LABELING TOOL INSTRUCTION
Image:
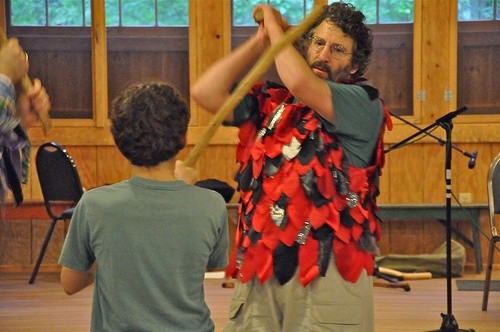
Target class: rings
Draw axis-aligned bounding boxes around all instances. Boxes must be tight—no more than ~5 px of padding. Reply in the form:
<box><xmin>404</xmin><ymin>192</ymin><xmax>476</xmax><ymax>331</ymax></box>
<box><xmin>24</xmin><ymin>52</ymin><xmax>28</xmax><ymax>62</ymax></box>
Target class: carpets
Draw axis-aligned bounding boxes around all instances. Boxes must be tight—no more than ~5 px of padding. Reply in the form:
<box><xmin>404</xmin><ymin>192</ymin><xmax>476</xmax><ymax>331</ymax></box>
<box><xmin>457</xmin><ymin>279</ymin><xmax>500</xmax><ymax>291</ymax></box>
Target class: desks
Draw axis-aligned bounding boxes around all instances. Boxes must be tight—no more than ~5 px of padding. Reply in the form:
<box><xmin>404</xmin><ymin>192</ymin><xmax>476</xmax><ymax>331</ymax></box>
<box><xmin>377</xmin><ymin>203</ymin><xmax>488</xmax><ymax>274</ymax></box>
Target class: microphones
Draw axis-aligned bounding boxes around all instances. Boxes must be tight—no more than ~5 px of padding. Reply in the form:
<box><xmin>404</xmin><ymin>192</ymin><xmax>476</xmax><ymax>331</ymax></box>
<box><xmin>469</xmin><ymin>150</ymin><xmax>478</xmax><ymax>168</ymax></box>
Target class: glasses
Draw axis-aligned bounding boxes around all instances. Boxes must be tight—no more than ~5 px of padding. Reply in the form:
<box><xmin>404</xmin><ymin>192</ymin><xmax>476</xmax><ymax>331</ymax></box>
<box><xmin>308</xmin><ymin>35</ymin><xmax>353</xmax><ymax>59</ymax></box>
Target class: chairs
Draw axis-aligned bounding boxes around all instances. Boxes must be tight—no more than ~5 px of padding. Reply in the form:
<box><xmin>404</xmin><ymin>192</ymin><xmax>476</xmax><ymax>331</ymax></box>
<box><xmin>29</xmin><ymin>141</ymin><xmax>83</xmax><ymax>284</ymax></box>
<box><xmin>480</xmin><ymin>153</ymin><xmax>500</xmax><ymax>312</ymax></box>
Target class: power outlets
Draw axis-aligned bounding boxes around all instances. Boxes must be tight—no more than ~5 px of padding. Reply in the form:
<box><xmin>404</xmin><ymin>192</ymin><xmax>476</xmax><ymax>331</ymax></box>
<box><xmin>458</xmin><ymin>193</ymin><xmax>471</xmax><ymax>204</ymax></box>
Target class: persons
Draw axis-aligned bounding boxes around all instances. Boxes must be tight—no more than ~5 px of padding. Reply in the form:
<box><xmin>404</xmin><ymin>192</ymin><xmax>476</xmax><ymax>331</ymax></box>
<box><xmin>58</xmin><ymin>81</ymin><xmax>229</xmax><ymax>332</ymax></box>
<box><xmin>190</xmin><ymin>1</ymin><xmax>393</xmax><ymax>332</ymax></box>
<box><xmin>0</xmin><ymin>37</ymin><xmax>51</xmax><ymax>208</ymax></box>
